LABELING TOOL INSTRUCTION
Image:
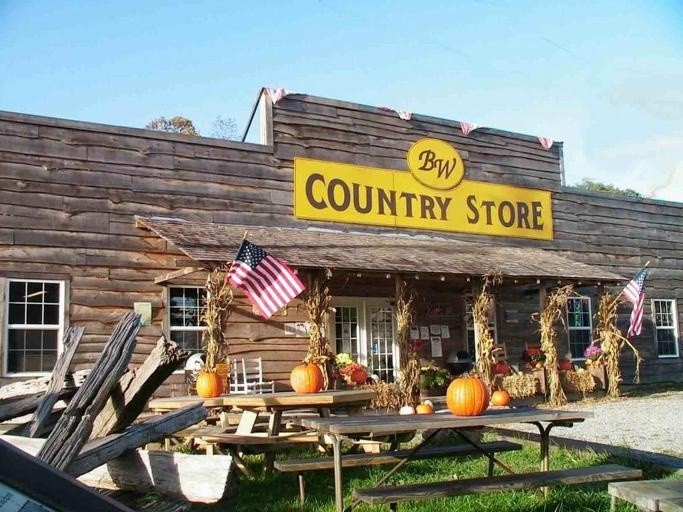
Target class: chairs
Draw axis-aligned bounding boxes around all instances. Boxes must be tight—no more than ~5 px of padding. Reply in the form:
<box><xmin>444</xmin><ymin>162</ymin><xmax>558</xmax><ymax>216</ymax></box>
<box><xmin>229</xmin><ymin>356</ymin><xmax>275</xmax><ymax>395</ymax></box>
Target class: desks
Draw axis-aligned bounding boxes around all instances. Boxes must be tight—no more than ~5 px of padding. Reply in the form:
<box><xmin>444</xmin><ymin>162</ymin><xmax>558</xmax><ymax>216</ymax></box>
<box><xmin>149</xmin><ymin>391</ymin><xmax>378</xmax><ymax>457</ymax></box>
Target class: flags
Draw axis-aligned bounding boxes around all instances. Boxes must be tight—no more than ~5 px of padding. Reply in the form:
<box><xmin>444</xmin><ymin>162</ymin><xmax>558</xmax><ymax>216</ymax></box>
<box><xmin>619</xmin><ymin>266</ymin><xmax>648</xmax><ymax>338</ymax></box>
<box><xmin>225</xmin><ymin>238</ymin><xmax>307</xmax><ymax>323</ymax></box>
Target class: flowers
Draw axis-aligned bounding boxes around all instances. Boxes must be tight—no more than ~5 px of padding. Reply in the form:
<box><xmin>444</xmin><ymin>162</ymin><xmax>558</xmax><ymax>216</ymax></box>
<box><xmin>527</xmin><ymin>346</ymin><xmax>545</xmax><ymax>362</ymax></box>
<box><xmin>584</xmin><ymin>345</ymin><xmax>601</xmax><ymax>361</ymax></box>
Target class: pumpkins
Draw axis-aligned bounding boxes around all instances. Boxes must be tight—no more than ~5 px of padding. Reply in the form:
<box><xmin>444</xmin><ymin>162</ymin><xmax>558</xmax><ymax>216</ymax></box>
<box><xmin>446</xmin><ymin>371</ymin><xmax>489</xmax><ymax>416</ymax></box>
<box><xmin>491</xmin><ymin>388</ymin><xmax>511</xmax><ymax>406</ymax></box>
<box><xmin>289</xmin><ymin>359</ymin><xmax>323</xmax><ymax>393</ymax></box>
<box><xmin>196</xmin><ymin>368</ymin><xmax>224</xmax><ymax>398</ymax></box>
<box><xmin>416</xmin><ymin>404</ymin><xmax>433</xmax><ymax>414</ymax></box>
<box><xmin>399</xmin><ymin>404</ymin><xmax>415</xmax><ymax>415</ymax></box>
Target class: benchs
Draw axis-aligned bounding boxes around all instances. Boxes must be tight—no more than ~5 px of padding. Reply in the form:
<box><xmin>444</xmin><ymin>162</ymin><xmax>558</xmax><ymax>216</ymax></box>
<box><xmin>272</xmin><ymin>440</ymin><xmax>644</xmax><ymax>507</ymax></box>
<box><xmin>163</xmin><ymin>410</ymin><xmax>354</xmax><ymax>446</ymax></box>
